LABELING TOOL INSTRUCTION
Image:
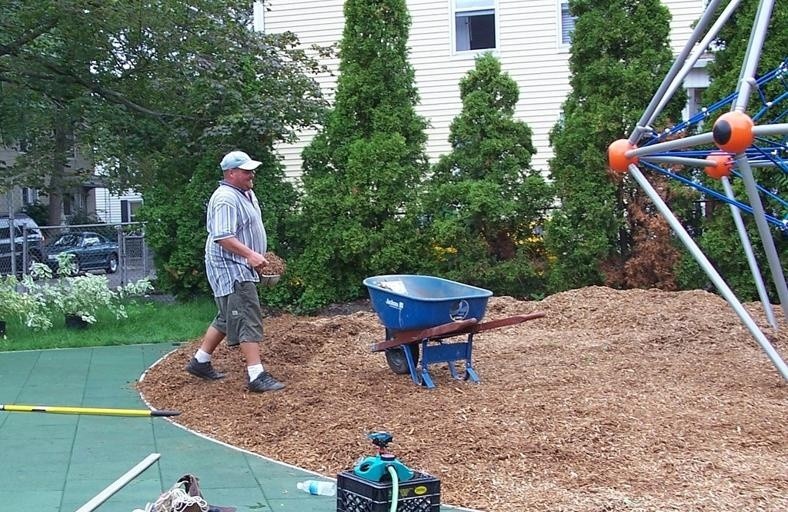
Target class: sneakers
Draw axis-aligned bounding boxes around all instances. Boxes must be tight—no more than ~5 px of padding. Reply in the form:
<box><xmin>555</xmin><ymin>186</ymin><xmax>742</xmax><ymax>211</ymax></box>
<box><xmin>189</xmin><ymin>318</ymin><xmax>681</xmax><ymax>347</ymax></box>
<box><xmin>248</xmin><ymin>370</ymin><xmax>286</xmax><ymax>392</ymax></box>
<box><xmin>184</xmin><ymin>356</ymin><xmax>224</xmax><ymax>381</ymax></box>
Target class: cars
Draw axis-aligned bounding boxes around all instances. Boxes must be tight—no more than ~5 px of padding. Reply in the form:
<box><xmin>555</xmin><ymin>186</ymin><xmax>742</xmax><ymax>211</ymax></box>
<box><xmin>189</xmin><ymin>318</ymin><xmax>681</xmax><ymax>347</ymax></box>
<box><xmin>0</xmin><ymin>213</ymin><xmax>119</xmax><ymax>281</ymax></box>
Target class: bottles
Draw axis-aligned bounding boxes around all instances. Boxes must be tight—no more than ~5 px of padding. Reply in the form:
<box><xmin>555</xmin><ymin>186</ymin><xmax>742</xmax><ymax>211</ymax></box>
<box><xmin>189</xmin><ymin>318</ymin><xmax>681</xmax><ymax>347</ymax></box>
<box><xmin>296</xmin><ymin>480</ymin><xmax>337</xmax><ymax>497</ymax></box>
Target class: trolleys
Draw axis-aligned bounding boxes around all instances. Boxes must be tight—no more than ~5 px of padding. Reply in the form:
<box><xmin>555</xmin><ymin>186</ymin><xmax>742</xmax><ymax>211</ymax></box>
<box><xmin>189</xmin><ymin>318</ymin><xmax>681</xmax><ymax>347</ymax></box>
<box><xmin>363</xmin><ymin>274</ymin><xmax>546</xmax><ymax>389</ymax></box>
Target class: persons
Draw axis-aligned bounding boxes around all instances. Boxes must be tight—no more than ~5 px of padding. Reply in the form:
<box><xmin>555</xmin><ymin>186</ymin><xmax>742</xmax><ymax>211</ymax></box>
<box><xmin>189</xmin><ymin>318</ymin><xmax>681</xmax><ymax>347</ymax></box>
<box><xmin>181</xmin><ymin>150</ymin><xmax>288</xmax><ymax>392</ymax></box>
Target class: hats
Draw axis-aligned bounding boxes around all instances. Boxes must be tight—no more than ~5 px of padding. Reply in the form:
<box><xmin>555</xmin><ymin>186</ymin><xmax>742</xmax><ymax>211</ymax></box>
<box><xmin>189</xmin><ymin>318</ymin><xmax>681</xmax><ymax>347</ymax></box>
<box><xmin>220</xmin><ymin>150</ymin><xmax>264</xmax><ymax>172</ymax></box>
